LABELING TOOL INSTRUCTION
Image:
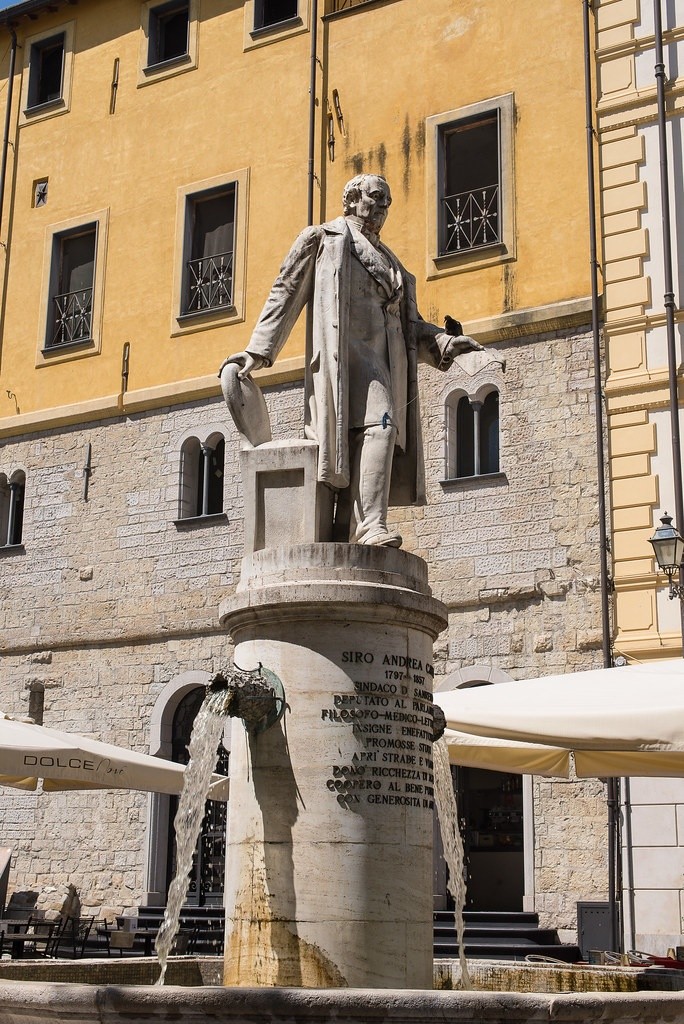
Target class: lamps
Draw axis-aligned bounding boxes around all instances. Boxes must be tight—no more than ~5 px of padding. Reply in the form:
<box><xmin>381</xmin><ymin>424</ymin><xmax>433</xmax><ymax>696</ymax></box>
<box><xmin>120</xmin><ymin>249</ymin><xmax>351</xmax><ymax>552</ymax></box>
<box><xmin>646</xmin><ymin>511</ymin><xmax>684</xmax><ymax>602</ymax></box>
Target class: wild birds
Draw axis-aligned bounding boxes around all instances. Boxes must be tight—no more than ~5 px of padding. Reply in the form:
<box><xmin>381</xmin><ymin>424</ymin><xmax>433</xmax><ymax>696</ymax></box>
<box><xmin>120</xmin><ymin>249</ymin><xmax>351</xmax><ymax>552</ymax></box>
<box><xmin>443</xmin><ymin>315</ymin><xmax>462</xmax><ymax>337</ymax></box>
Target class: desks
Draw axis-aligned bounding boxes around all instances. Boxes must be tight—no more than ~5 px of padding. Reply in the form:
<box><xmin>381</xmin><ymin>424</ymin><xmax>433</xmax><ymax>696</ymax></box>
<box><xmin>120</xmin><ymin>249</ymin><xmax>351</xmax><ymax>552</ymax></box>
<box><xmin>0</xmin><ymin>934</ymin><xmax>60</xmax><ymax>959</ymax></box>
<box><xmin>96</xmin><ymin>928</ymin><xmax>160</xmax><ymax>958</ymax></box>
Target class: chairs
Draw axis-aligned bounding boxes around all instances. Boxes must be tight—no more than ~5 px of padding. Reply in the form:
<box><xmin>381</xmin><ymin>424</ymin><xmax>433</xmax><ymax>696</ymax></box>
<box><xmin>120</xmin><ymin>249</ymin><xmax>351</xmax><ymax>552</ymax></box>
<box><xmin>627</xmin><ymin>950</ymin><xmax>656</xmax><ymax>966</ymax></box>
<box><xmin>604</xmin><ymin>950</ymin><xmax>639</xmax><ymax>966</ymax></box>
<box><xmin>21</xmin><ymin>916</ymin><xmax>63</xmax><ymax>960</ymax></box>
<box><xmin>525</xmin><ymin>954</ymin><xmax>570</xmax><ymax>965</ymax></box>
<box><xmin>169</xmin><ymin>926</ymin><xmax>200</xmax><ymax>955</ymax></box>
<box><xmin>72</xmin><ymin>918</ymin><xmax>110</xmax><ymax>959</ymax></box>
<box><xmin>54</xmin><ymin>916</ymin><xmax>94</xmax><ymax>959</ymax></box>
